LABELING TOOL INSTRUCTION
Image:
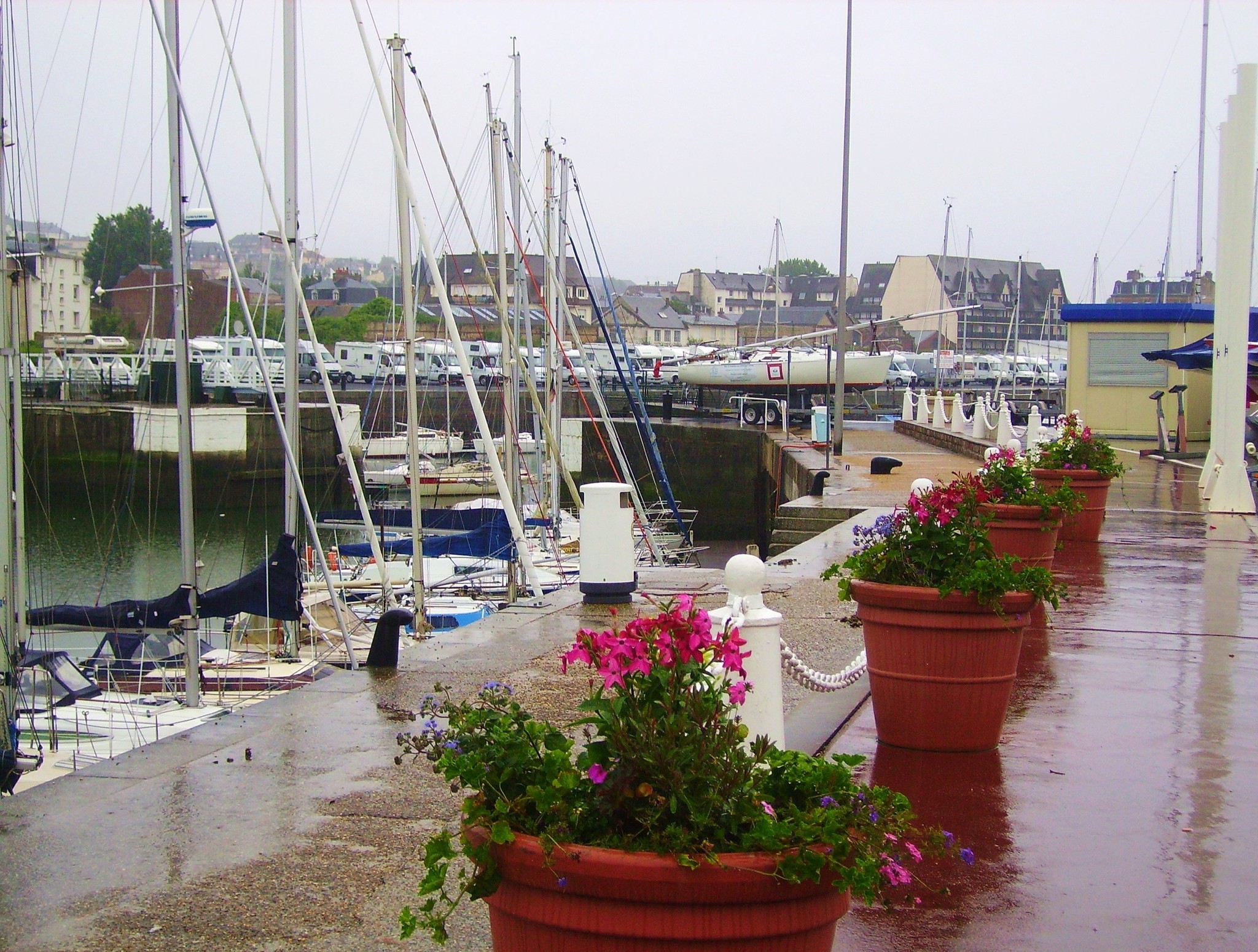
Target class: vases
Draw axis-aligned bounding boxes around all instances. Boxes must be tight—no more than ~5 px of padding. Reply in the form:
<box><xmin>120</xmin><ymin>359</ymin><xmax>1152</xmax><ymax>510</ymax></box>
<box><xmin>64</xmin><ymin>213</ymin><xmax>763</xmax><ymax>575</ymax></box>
<box><xmin>848</xmin><ymin>576</ymin><xmax>1037</xmax><ymax>751</ymax></box>
<box><xmin>975</xmin><ymin>498</ymin><xmax>1061</xmax><ymax>588</ymax></box>
<box><xmin>1032</xmin><ymin>462</ymin><xmax>1111</xmax><ymax>546</ymax></box>
<box><xmin>460</xmin><ymin>794</ymin><xmax>852</xmax><ymax>952</ymax></box>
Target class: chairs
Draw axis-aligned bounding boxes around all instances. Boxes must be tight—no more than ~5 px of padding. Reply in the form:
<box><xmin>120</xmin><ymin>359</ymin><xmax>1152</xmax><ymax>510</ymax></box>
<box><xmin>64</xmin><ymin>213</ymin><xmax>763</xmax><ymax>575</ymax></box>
<box><xmin>1008</xmin><ymin>400</ymin><xmax>1028</xmax><ymax>426</ymax></box>
<box><xmin>1038</xmin><ymin>401</ymin><xmax>1059</xmax><ymax>428</ymax></box>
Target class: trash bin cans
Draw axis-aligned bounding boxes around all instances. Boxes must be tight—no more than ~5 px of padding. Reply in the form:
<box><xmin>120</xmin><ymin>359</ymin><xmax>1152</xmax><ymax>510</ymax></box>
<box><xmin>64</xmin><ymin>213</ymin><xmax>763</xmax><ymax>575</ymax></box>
<box><xmin>811</xmin><ymin>406</ymin><xmax>830</xmax><ymax>444</ymax></box>
<box><xmin>579</xmin><ymin>483</ymin><xmax>639</xmax><ymax>603</ymax></box>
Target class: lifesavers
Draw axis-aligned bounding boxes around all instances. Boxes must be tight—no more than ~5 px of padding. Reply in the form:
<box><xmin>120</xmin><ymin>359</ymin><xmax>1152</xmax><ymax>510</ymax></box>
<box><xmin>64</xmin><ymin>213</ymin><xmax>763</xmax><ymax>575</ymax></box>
<box><xmin>305</xmin><ymin>546</ymin><xmax>312</xmax><ymax>572</ymax></box>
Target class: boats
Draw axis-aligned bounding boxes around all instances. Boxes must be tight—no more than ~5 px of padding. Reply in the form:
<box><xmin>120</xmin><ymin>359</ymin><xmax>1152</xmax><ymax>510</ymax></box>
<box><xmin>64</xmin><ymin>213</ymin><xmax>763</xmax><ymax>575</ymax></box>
<box><xmin>675</xmin><ymin>218</ymin><xmax>900</xmax><ymax>396</ymax></box>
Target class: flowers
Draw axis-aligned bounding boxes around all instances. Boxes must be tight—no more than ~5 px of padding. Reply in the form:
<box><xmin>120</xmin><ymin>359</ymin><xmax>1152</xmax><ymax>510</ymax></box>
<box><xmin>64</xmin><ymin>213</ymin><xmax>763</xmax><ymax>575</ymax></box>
<box><xmin>388</xmin><ymin>594</ymin><xmax>971</xmax><ymax>931</ymax></box>
<box><xmin>1034</xmin><ymin>414</ymin><xmax>1138</xmax><ymax>518</ymax></box>
<box><xmin>817</xmin><ymin>479</ymin><xmax>1060</xmax><ymax>612</ymax></box>
<box><xmin>955</xmin><ymin>453</ymin><xmax>1087</xmax><ymax>514</ymax></box>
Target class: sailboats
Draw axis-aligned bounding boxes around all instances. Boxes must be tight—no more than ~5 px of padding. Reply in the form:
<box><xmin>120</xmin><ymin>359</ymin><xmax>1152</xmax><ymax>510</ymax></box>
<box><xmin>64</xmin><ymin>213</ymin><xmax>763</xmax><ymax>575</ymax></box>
<box><xmin>0</xmin><ymin>0</ymin><xmax>1067</xmax><ymax>808</ymax></box>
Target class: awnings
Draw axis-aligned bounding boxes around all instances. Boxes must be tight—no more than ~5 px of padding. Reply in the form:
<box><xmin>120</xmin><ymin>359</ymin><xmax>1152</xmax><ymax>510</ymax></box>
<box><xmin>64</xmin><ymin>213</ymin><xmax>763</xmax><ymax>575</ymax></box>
<box><xmin>1141</xmin><ymin>312</ymin><xmax>1258</xmax><ymax>370</ymax></box>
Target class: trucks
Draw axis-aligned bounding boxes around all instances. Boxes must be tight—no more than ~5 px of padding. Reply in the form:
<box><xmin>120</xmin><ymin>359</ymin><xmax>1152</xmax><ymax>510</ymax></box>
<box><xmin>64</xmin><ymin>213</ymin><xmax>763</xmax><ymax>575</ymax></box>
<box><xmin>972</xmin><ymin>355</ymin><xmax>1006</xmax><ymax>386</ymax></box>
<box><xmin>461</xmin><ymin>339</ymin><xmax>508</xmax><ymax>386</ymax></box>
<box><xmin>276</xmin><ymin>339</ymin><xmax>343</xmax><ymax>385</ymax></box>
<box><xmin>191</xmin><ymin>334</ymin><xmax>286</xmax><ymax>388</ymax></box>
<box><xmin>415</xmin><ymin>338</ymin><xmax>465</xmax><ymax>386</ymax></box>
<box><xmin>514</xmin><ymin>346</ymin><xmax>548</xmax><ymax>388</ymax></box>
<box><xmin>557</xmin><ymin>340</ymin><xmax>597</xmax><ymax>387</ymax></box>
<box><xmin>997</xmin><ymin>356</ymin><xmax>1035</xmax><ymax>386</ymax></box>
<box><xmin>1025</xmin><ymin>357</ymin><xmax>1059</xmax><ymax>385</ymax></box>
<box><xmin>142</xmin><ymin>336</ymin><xmax>234</xmax><ymax>384</ymax></box>
<box><xmin>582</xmin><ymin>342</ymin><xmax>664</xmax><ymax>387</ymax></box>
<box><xmin>1045</xmin><ymin>358</ymin><xmax>1067</xmax><ymax>383</ymax></box>
<box><xmin>44</xmin><ymin>328</ymin><xmax>137</xmax><ymax>387</ymax></box>
<box><xmin>333</xmin><ymin>340</ymin><xmax>419</xmax><ymax>386</ymax></box>
